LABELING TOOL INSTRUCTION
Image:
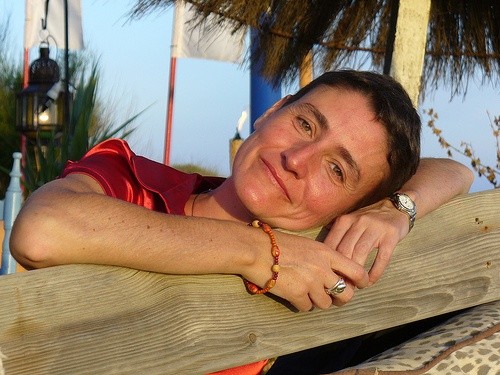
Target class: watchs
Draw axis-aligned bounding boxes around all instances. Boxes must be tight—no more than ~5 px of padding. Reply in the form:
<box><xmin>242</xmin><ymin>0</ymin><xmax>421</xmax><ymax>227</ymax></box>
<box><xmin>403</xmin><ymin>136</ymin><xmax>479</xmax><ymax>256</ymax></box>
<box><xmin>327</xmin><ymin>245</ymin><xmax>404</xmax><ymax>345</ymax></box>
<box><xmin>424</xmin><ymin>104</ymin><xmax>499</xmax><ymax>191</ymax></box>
<box><xmin>389</xmin><ymin>191</ymin><xmax>416</xmax><ymax>232</ymax></box>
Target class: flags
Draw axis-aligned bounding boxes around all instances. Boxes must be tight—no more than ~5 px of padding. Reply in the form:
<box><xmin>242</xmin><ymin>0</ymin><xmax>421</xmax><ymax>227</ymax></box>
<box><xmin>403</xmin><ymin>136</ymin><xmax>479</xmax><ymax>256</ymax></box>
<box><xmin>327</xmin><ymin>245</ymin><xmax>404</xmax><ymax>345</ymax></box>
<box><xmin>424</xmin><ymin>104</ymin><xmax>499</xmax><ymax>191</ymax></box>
<box><xmin>177</xmin><ymin>0</ymin><xmax>244</xmax><ymax>62</ymax></box>
<box><xmin>26</xmin><ymin>0</ymin><xmax>84</xmax><ymax>51</ymax></box>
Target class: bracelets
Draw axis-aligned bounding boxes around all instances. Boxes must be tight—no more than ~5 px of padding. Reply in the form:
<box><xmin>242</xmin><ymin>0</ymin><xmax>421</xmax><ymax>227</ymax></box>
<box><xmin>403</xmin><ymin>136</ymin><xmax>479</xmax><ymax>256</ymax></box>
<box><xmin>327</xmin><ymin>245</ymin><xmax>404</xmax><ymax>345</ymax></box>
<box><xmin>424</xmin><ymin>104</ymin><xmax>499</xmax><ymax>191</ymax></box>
<box><xmin>242</xmin><ymin>220</ymin><xmax>280</xmax><ymax>295</ymax></box>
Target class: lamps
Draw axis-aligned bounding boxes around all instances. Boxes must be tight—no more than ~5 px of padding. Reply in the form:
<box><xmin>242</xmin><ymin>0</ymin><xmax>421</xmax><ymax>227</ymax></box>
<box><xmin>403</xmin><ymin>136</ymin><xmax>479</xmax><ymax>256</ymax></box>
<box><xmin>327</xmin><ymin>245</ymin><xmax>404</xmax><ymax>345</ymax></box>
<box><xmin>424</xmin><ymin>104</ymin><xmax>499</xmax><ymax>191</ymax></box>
<box><xmin>14</xmin><ymin>0</ymin><xmax>74</xmax><ymax>146</ymax></box>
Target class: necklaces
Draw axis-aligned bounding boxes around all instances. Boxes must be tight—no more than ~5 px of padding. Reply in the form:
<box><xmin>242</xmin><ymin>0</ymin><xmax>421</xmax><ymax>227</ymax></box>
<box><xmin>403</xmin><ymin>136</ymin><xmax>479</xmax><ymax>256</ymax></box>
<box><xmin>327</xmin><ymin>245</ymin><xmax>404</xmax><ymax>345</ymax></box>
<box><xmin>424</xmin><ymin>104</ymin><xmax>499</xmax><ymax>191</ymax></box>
<box><xmin>192</xmin><ymin>188</ymin><xmax>213</xmax><ymax>216</ymax></box>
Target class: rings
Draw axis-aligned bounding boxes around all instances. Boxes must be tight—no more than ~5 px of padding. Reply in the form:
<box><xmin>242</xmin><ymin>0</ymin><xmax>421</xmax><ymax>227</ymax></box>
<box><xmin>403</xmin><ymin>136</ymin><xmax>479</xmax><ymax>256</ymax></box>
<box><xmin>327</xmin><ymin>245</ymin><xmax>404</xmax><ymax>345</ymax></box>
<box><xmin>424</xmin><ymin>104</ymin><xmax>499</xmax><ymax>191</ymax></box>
<box><xmin>325</xmin><ymin>276</ymin><xmax>346</xmax><ymax>295</ymax></box>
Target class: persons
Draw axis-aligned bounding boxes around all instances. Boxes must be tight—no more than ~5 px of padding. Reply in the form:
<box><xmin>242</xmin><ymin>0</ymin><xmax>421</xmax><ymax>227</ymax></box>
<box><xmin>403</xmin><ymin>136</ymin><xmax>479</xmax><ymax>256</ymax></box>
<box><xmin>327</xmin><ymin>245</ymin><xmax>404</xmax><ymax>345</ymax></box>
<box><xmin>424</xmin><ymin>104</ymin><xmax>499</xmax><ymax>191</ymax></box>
<box><xmin>10</xmin><ymin>70</ymin><xmax>474</xmax><ymax>375</ymax></box>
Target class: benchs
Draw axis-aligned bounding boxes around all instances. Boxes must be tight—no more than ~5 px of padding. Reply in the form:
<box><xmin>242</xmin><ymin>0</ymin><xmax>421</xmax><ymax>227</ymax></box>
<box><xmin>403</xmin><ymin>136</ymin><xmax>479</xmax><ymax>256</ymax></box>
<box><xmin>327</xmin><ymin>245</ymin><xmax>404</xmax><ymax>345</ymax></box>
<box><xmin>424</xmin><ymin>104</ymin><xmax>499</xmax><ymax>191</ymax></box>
<box><xmin>0</xmin><ymin>188</ymin><xmax>500</xmax><ymax>375</ymax></box>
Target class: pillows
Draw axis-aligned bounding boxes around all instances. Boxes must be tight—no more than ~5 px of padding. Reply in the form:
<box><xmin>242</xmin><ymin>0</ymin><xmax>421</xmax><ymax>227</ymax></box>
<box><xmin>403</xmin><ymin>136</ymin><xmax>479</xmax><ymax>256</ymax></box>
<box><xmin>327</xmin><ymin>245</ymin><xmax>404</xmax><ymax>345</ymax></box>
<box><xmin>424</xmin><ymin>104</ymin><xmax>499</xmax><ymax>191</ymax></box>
<box><xmin>323</xmin><ymin>301</ymin><xmax>500</xmax><ymax>375</ymax></box>
